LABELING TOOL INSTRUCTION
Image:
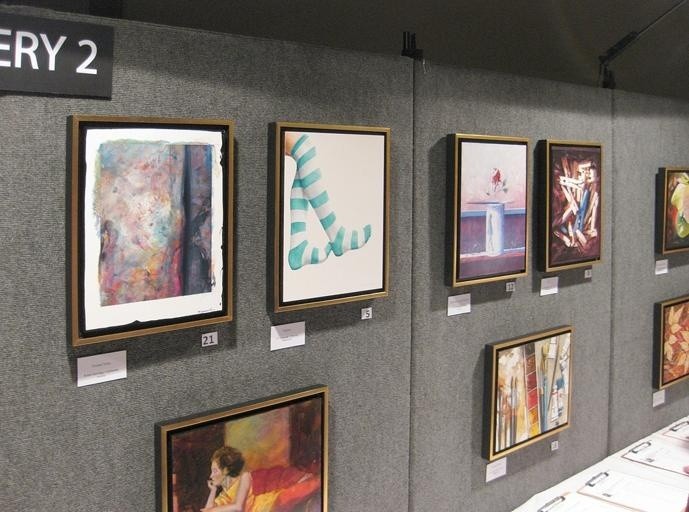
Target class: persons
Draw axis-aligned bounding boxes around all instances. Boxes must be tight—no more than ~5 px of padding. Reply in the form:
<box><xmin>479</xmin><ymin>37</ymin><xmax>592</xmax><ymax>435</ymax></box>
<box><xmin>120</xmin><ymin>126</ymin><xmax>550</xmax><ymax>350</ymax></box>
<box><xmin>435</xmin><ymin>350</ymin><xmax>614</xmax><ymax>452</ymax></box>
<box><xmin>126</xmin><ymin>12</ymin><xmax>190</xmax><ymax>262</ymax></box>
<box><xmin>282</xmin><ymin>129</ymin><xmax>372</xmax><ymax>271</ymax></box>
<box><xmin>199</xmin><ymin>444</ymin><xmax>279</xmax><ymax>512</ymax></box>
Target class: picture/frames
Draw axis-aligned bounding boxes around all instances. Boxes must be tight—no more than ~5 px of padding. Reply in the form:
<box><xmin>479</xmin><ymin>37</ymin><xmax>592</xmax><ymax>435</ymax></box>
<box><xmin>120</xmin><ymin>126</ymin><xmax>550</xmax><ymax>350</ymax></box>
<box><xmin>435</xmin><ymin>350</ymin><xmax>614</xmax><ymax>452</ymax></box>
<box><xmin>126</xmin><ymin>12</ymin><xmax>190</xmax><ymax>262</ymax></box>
<box><xmin>65</xmin><ymin>115</ymin><xmax>236</xmax><ymax>347</ymax></box>
<box><xmin>542</xmin><ymin>139</ymin><xmax>604</xmax><ymax>274</ymax></box>
<box><xmin>653</xmin><ymin>165</ymin><xmax>688</xmax><ymax>256</ymax></box>
<box><xmin>267</xmin><ymin>121</ymin><xmax>391</xmax><ymax>312</ymax></box>
<box><xmin>483</xmin><ymin>323</ymin><xmax>573</xmax><ymax>462</ymax></box>
<box><xmin>446</xmin><ymin>133</ymin><xmax>531</xmax><ymax>289</ymax></box>
<box><xmin>151</xmin><ymin>383</ymin><xmax>332</xmax><ymax>511</ymax></box>
<box><xmin>652</xmin><ymin>292</ymin><xmax>689</xmax><ymax>392</ymax></box>
